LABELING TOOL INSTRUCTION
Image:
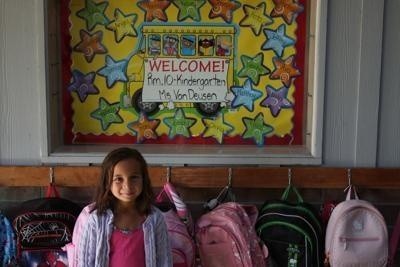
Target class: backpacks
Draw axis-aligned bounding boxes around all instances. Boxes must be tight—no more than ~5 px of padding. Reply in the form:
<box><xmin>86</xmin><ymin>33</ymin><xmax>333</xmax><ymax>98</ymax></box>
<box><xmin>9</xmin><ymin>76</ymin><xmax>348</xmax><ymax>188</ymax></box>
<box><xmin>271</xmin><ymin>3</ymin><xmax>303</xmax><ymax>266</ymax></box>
<box><xmin>153</xmin><ymin>182</ymin><xmax>200</xmax><ymax>267</ymax></box>
<box><xmin>11</xmin><ymin>184</ymin><xmax>81</xmax><ymax>267</ymax></box>
<box><xmin>193</xmin><ymin>184</ymin><xmax>274</xmax><ymax>267</ymax></box>
<box><xmin>256</xmin><ymin>182</ymin><xmax>325</xmax><ymax>267</ymax></box>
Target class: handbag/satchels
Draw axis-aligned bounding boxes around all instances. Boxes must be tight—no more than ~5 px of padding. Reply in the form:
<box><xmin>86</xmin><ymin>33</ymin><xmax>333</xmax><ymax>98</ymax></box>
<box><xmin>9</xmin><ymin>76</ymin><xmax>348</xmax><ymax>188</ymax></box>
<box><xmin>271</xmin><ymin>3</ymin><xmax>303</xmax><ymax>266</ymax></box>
<box><xmin>325</xmin><ymin>186</ymin><xmax>389</xmax><ymax>267</ymax></box>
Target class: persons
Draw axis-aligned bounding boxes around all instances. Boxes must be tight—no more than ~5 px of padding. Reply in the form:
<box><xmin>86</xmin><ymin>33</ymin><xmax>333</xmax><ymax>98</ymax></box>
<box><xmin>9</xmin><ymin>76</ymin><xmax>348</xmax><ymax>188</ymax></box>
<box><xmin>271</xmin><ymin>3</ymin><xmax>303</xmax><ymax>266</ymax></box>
<box><xmin>65</xmin><ymin>146</ymin><xmax>174</xmax><ymax>267</ymax></box>
